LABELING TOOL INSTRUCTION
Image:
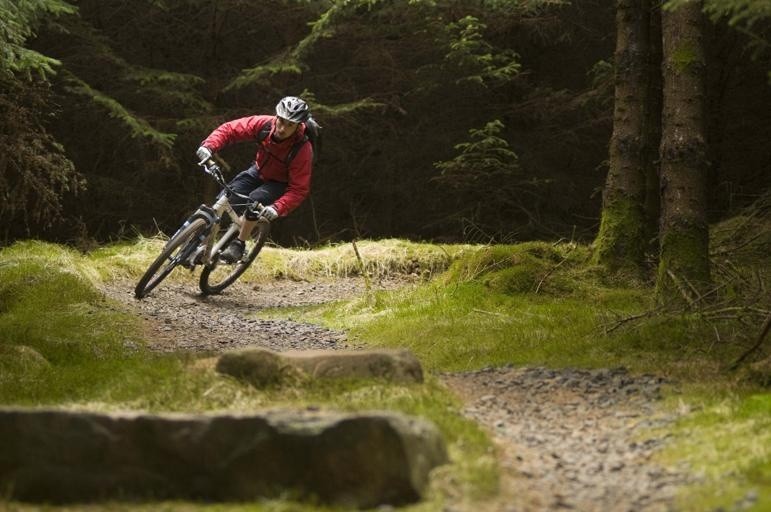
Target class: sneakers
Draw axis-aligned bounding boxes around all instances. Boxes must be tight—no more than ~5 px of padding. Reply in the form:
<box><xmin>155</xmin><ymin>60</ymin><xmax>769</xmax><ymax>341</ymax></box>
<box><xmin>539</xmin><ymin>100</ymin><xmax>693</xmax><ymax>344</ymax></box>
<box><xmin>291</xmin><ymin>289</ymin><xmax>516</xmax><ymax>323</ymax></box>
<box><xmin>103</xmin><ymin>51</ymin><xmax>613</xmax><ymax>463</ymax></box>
<box><xmin>221</xmin><ymin>240</ymin><xmax>246</xmax><ymax>263</ymax></box>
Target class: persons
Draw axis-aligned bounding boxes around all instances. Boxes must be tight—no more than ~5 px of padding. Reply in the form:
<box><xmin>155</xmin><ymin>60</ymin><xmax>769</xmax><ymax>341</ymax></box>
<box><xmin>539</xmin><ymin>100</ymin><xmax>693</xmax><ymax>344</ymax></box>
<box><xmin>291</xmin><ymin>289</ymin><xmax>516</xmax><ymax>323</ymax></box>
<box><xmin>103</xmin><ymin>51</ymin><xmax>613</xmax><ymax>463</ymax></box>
<box><xmin>186</xmin><ymin>95</ymin><xmax>313</xmax><ymax>266</ymax></box>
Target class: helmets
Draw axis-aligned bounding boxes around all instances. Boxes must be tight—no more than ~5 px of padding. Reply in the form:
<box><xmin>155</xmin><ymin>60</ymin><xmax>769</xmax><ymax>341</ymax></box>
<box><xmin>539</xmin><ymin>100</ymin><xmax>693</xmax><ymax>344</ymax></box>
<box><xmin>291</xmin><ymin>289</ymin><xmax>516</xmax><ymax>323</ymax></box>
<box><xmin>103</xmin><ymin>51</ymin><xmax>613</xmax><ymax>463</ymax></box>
<box><xmin>276</xmin><ymin>96</ymin><xmax>312</xmax><ymax>124</ymax></box>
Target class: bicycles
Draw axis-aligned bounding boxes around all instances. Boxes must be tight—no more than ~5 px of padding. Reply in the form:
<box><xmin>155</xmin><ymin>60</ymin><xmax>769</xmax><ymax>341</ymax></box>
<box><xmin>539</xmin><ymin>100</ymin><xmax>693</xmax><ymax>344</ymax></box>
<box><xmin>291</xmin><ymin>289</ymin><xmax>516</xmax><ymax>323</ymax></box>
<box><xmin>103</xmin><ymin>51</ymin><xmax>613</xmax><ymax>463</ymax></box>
<box><xmin>133</xmin><ymin>158</ymin><xmax>272</xmax><ymax>300</ymax></box>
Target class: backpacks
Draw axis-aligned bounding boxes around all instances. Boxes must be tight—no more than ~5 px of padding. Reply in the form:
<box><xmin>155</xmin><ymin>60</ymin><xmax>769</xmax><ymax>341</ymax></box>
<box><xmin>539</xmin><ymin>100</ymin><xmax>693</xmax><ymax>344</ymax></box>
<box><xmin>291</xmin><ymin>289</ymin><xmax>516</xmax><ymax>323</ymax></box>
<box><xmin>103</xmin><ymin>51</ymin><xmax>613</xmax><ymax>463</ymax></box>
<box><xmin>258</xmin><ymin>114</ymin><xmax>324</xmax><ymax>171</ymax></box>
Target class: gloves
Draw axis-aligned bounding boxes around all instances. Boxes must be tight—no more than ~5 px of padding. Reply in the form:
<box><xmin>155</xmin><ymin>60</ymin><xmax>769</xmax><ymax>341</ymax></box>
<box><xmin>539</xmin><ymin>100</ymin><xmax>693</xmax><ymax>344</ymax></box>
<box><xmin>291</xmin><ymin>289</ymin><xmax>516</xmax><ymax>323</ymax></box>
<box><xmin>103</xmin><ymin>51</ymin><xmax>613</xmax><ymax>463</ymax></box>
<box><xmin>195</xmin><ymin>146</ymin><xmax>214</xmax><ymax>167</ymax></box>
<box><xmin>257</xmin><ymin>206</ymin><xmax>280</xmax><ymax>223</ymax></box>
